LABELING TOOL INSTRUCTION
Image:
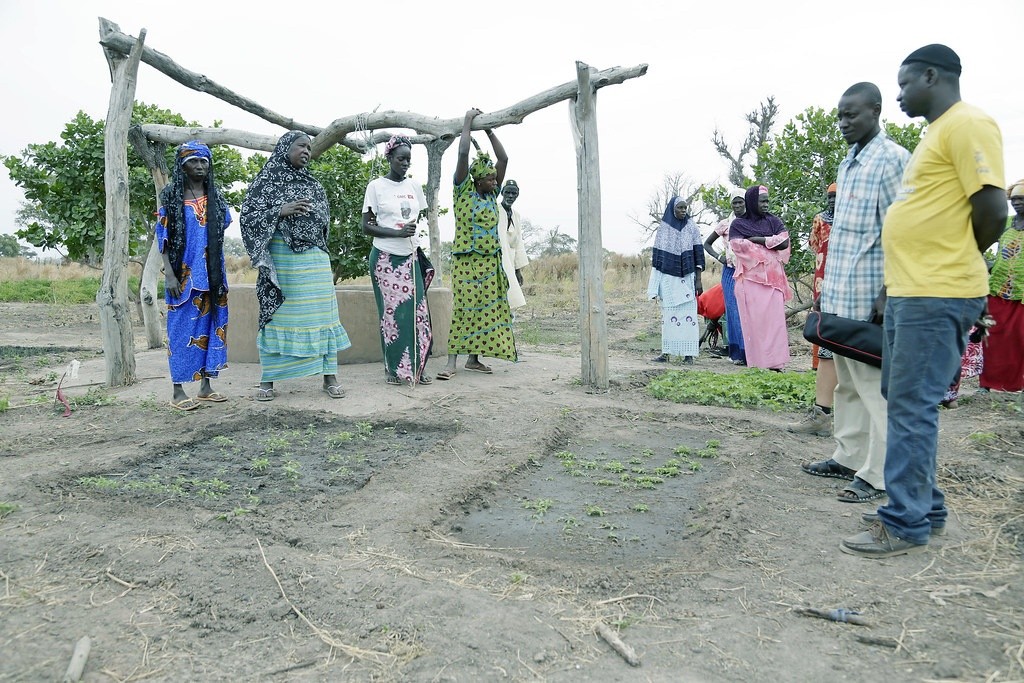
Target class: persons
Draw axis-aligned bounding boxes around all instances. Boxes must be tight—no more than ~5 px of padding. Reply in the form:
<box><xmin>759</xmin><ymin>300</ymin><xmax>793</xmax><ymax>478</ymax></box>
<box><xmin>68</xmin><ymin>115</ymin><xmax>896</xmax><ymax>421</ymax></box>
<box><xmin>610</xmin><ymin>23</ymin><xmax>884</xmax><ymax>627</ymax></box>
<box><xmin>437</xmin><ymin>107</ymin><xmax>518</xmax><ymax>380</ymax></box>
<box><xmin>155</xmin><ymin>139</ymin><xmax>234</xmax><ymax>410</ymax></box>
<box><xmin>728</xmin><ymin>186</ymin><xmax>791</xmax><ymax>372</ymax></box>
<box><xmin>940</xmin><ymin>179</ymin><xmax>1024</xmax><ymax>409</ymax></box>
<box><xmin>498</xmin><ymin>180</ymin><xmax>529</xmax><ymax>311</ymax></box>
<box><xmin>239</xmin><ymin>130</ymin><xmax>352</xmax><ymax>400</ymax></box>
<box><xmin>647</xmin><ymin>196</ymin><xmax>705</xmax><ymax>365</ymax></box>
<box><xmin>704</xmin><ymin>189</ymin><xmax>747</xmax><ymax>365</ymax></box>
<box><xmin>808</xmin><ymin>183</ymin><xmax>837</xmax><ymax>369</ymax></box>
<box><xmin>839</xmin><ymin>43</ymin><xmax>1008</xmax><ymax>559</ymax></box>
<box><xmin>787</xmin><ymin>345</ymin><xmax>837</xmax><ymax>437</ymax></box>
<box><xmin>361</xmin><ymin>133</ymin><xmax>435</xmax><ymax>387</ymax></box>
<box><xmin>800</xmin><ymin>81</ymin><xmax>912</xmax><ymax>502</ymax></box>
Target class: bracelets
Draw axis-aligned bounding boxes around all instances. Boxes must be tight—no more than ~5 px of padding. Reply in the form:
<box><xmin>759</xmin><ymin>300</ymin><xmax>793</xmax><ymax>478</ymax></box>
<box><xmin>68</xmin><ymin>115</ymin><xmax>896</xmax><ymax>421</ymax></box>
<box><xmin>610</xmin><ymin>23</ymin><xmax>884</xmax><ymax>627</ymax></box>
<box><xmin>717</xmin><ymin>255</ymin><xmax>722</xmax><ymax>260</ymax></box>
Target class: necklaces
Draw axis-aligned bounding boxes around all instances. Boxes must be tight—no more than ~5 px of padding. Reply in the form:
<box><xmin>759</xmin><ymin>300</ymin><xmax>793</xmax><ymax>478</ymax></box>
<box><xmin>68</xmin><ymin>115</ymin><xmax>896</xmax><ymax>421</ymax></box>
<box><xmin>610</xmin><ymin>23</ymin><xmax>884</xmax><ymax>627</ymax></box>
<box><xmin>1012</xmin><ymin>216</ymin><xmax>1024</xmax><ymax>231</ymax></box>
<box><xmin>187</xmin><ymin>182</ymin><xmax>206</xmax><ymax>220</ymax></box>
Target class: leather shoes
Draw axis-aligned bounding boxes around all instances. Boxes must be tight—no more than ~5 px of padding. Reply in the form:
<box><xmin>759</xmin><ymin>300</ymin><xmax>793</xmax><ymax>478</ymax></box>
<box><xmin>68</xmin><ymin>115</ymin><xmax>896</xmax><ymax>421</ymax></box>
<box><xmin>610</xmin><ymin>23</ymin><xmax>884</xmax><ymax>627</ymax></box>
<box><xmin>858</xmin><ymin>511</ymin><xmax>946</xmax><ymax>535</ymax></box>
<box><xmin>839</xmin><ymin>518</ymin><xmax>927</xmax><ymax>559</ymax></box>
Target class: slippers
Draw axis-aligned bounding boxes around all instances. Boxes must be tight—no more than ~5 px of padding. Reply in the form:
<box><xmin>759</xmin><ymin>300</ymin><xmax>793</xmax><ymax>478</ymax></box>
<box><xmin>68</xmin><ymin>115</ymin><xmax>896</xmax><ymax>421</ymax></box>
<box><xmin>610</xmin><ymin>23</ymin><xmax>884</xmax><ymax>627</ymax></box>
<box><xmin>419</xmin><ymin>375</ymin><xmax>433</xmax><ymax>384</ymax></box>
<box><xmin>196</xmin><ymin>391</ymin><xmax>228</xmax><ymax>401</ymax></box>
<box><xmin>437</xmin><ymin>370</ymin><xmax>455</xmax><ymax>379</ymax></box>
<box><xmin>322</xmin><ymin>385</ymin><xmax>345</xmax><ymax>397</ymax></box>
<box><xmin>170</xmin><ymin>398</ymin><xmax>201</xmax><ymax>410</ymax></box>
<box><xmin>258</xmin><ymin>388</ymin><xmax>273</xmax><ymax>400</ymax></box>
<box><xmin>383</xmin><ymin>375</ymin><xmax>402</xmax><ymax>384</ymax></box>
<box><xmin>464</xmin><ymin>363</ymin><xmax>492</xmax><ymax>373</ymax></box>
<box><xmin>800</xmin><ymin>459</ymin><xmax>857</xmax><ymax>480</ymax></box>
<box><xmin>835</xmin><ymin>478</ymin><xmax>887</xmax><ymax>502</ymax></box>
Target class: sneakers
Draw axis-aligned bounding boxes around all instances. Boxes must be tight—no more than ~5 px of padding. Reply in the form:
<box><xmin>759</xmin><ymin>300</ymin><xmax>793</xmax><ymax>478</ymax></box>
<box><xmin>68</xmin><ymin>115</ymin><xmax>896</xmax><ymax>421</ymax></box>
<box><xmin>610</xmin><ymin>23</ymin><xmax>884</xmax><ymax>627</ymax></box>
<box><xmin>787</xmin><ymin>403</ymin><xmax>831</xmax><ymax>436</ymax></box>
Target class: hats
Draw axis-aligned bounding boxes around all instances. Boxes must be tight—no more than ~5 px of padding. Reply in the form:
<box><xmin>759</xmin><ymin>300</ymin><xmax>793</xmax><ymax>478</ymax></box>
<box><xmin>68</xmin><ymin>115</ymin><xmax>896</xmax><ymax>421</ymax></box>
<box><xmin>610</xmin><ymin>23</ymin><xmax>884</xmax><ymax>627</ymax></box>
<box><xmin>469</xmin><ymin>150</ymin><xmax>497</xmax><ymax>178</ymax></box>
<box><xmin>904</xmin><ymin>43</ymin><xmax>960</xmax><ymax>76</ymax></box>
<box><xmin>502</xmin><ymin>179</ymin><xmax>519</xmax><ymax>190</ymax></box>
<box><xmin>385</xmin><ymin>132</ymin><xmax>411</xmax><ymax>152</ymax></box>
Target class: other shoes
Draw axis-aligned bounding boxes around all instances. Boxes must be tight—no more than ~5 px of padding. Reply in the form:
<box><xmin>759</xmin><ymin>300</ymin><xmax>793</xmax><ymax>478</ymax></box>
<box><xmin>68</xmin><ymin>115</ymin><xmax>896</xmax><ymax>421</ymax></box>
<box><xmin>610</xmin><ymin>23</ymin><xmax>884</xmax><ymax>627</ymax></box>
<box><xmin>683</xmin><ymin>358</ymin><xmax>693</xmax><ymax>365</ymax></box>
<box><xmin>732</xmin><ymin>359</ymin><xmax>746</xmax><ymax>365</ymax></box>
<box><xmin>652</xmin><ymin>355</ymin><xmax>669</xmax><ymax>362</ymax></box>
<box><xmin>944</xmin><ymin>400</ymin><xmax>959</xmax><ymax>408</ymax></box>
